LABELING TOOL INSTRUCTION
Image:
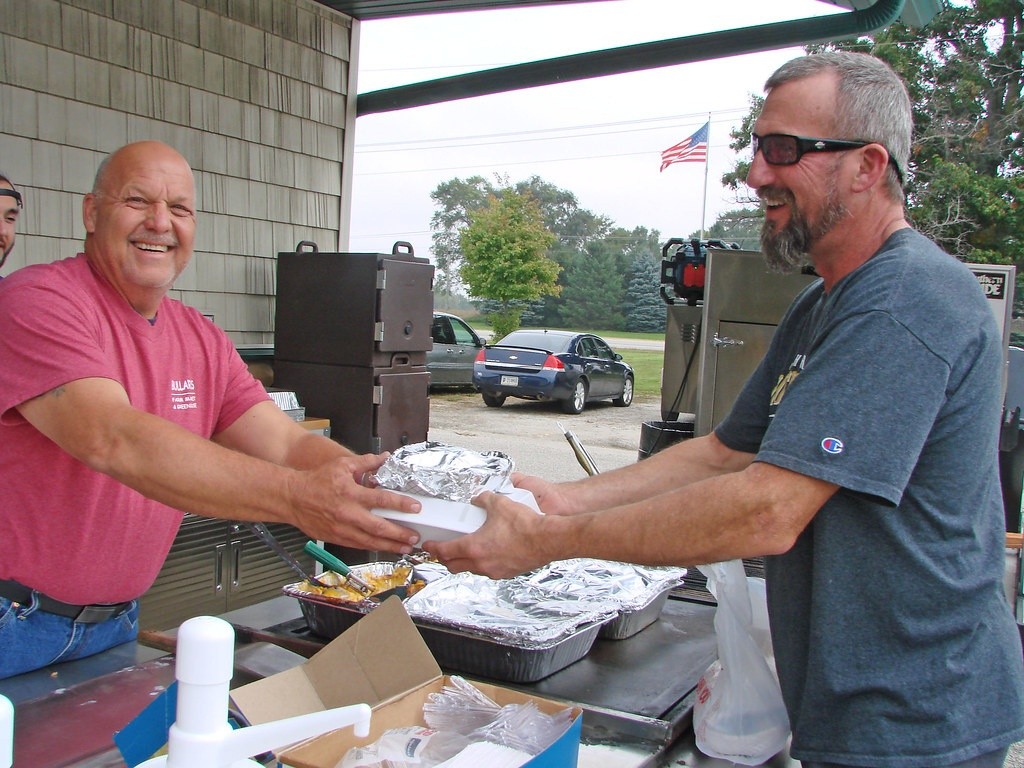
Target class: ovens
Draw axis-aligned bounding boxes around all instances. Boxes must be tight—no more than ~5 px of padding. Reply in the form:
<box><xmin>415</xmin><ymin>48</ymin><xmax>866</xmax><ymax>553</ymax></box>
<box><xmin>273</xmin><ymin>239</ymin><xmax>434</xmax><ymax>460</ymax></box>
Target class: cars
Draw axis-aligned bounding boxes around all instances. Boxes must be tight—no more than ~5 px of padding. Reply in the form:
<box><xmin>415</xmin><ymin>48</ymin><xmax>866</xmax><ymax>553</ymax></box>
<box><xmin>426</xmin><ymin>312</ymin><xmax>488</xmax><ymax>395</ymax></box>
<box><xmin>472</xmin><ymin>329</ymin><xmax>634</xmax><ymax>415</ymax></box>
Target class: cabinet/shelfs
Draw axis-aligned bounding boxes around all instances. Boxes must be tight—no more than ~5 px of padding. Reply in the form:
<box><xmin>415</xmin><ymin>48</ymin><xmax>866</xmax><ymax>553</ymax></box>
<box><xmin>273</xmin><ymin>239</ymin><xmax>434</xmax><ymax>457</ymax></box>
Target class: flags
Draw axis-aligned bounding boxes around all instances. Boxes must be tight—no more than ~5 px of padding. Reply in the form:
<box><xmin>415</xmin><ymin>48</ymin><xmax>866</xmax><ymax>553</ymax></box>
<box><xmin>654</xmin><ymin>121</ymin><xmax>711</xmax><ymax>174</ymax></box>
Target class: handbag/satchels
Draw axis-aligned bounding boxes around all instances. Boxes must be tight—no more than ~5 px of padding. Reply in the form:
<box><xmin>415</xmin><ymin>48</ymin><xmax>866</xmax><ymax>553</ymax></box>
<box><xmin>691</xmin><ymin>559</ymin><xmax>792</xmax><ymax>765</ymax></box>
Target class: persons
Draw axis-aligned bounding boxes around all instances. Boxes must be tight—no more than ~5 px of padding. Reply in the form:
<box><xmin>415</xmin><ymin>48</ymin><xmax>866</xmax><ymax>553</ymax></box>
<box><xmin>0</xmin><ymin>175</ymin><xmax>22</xmax><ymax>268</ymax></box>
<box><xmin>420</xmin><ymin>52</ymin><xmax>1024</xmax><ymax>767</ymax></box>
<box><xmin>0</xmin><ymin>138</ymin><xmax>422</xmax><ymax>680</ymax></box>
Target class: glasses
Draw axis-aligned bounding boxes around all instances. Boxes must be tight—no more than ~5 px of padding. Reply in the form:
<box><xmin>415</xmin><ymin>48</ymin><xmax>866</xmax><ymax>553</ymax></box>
<box><xmin>751</xmin><ymin>133</ymin><xmax>904</xmax><ymax>186</ymax></box>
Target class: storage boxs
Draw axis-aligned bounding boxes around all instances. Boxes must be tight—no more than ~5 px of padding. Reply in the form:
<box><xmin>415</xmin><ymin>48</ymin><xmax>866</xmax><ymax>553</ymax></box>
<box><xmin>228</xmin><ymin>596</ymin><xmax>583</xmax><ymax>767</ymax></box>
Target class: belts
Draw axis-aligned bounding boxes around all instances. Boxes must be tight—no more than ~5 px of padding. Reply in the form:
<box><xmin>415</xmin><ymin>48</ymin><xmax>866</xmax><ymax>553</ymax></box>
<box><xmin>0</xmin><ymin>579</ymin><xmax>130</xmax><ymax>623</ymax></box>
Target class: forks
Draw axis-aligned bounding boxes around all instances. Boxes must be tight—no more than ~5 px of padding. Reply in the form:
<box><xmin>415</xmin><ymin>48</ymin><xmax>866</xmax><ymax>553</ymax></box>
<box><xmin>421</xmin><ymin>673</ymin><xmax>574</xmax><ymax>756</ymax></box>
